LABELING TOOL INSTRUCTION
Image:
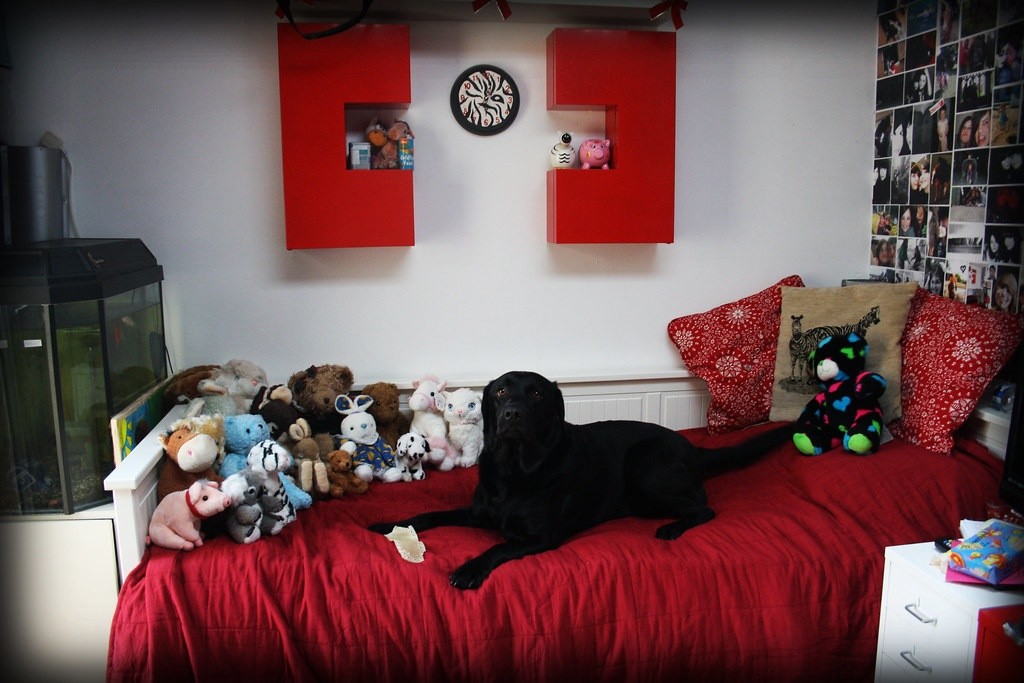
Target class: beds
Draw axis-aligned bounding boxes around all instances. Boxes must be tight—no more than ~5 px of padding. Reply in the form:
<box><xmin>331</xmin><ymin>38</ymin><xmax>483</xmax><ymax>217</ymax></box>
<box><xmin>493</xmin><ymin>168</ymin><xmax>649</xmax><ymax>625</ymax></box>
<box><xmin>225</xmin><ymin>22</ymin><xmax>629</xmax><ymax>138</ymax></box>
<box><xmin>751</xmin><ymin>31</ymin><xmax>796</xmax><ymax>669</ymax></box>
<box><xmin>102</xmin><ymin>404</ymin><xmax>1009</xmax><ymax>683</ymax></box>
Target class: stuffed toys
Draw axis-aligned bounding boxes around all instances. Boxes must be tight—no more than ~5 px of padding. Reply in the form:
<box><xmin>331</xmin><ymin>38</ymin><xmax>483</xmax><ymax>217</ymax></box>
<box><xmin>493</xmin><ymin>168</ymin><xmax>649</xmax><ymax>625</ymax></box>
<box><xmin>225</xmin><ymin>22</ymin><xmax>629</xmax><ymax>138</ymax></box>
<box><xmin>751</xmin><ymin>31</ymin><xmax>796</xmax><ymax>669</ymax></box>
<box><xmin>790</xmin><ymin>332</ymin><xmax>887</xmax><ymax>455</ymax></box>
<box><xmin>364</xmin><ymin>115</ymin><xmax>415</xmax><ymax>170</ymax></box>
<box><xmin>147</xmin><ymin>360</ymin><xmax>486</xmax><ymax>550</ymax></box>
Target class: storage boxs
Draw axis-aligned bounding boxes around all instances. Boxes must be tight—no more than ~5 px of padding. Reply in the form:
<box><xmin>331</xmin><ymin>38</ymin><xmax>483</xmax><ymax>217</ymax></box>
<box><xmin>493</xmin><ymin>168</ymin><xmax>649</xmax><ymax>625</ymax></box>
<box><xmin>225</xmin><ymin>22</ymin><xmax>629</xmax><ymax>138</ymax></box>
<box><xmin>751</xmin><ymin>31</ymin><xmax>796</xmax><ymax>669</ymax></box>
<box><xmin>0</xmin><ymin>238</ymin><xmax>168</xmax><ymax>516</ymax></box>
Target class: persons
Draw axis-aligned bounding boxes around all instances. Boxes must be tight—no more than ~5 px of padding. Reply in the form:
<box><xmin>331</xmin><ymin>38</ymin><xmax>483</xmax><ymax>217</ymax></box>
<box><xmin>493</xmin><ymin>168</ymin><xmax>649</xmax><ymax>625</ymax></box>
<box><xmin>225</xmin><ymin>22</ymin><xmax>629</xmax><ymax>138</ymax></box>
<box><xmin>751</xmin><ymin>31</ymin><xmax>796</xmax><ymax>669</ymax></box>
<box><xmin>870</xmin><ymin>264</ymin><xmax>1017</xmax><ymax>316</ymax></box>
<box><xmin>872</xmin><ymin>151</ymin><xmax>1024</xmax><ymax>265</ymax></box>
<box><xmin>875</xmin><ymin>103</ymin><xmax>1024</xmax><ymax>158</ymax></box>
<box><xmin>877</xmin><ymin>0</ymin><xmax>1024</xmax><ymax>111</ymax></box>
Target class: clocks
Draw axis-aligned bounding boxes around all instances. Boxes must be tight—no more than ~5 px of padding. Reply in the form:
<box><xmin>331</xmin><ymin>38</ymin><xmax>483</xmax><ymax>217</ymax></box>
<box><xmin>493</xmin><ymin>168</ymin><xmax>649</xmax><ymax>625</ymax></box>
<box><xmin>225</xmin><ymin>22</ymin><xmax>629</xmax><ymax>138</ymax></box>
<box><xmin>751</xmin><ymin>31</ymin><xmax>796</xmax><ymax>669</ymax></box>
<box><xmin>449</xmin><ymin>65</ymin><xmax>521</xmax><ymax>137</ymax></box>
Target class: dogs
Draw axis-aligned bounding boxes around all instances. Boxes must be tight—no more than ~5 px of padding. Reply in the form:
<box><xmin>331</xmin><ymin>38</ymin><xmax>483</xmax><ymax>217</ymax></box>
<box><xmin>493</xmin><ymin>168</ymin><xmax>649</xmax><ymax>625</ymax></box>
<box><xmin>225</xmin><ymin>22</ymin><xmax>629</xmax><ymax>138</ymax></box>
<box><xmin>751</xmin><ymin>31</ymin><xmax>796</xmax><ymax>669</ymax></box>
<box><xmin>364</xmin><ymin>372</ymin><xmax>798</xmax><ymax>590</ymax></box>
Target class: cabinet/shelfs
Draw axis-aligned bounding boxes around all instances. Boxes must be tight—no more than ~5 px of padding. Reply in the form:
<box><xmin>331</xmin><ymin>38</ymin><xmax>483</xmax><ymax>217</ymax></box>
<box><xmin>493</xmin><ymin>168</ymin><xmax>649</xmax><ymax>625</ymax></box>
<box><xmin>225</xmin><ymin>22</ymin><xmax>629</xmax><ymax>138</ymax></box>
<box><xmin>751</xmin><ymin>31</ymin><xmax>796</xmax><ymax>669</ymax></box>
<box><xmin>0</xmin><ymin>502</ymin><xmax>121</xmax><ymax>683</ymax></box>
<box><xmin>875</xmin><ymin>537</ymin><xmax>1024</xmax><ymax>683</ymax></box>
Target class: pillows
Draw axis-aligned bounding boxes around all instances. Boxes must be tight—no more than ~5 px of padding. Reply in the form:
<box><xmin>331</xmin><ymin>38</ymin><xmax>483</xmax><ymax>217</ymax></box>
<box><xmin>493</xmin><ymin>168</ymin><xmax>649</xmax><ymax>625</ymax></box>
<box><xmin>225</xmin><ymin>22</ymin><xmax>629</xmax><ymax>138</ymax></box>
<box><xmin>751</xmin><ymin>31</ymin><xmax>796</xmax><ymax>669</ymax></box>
<box><xmin>668</xmin><ymin>274</ymin><xmax>804</xmax><ymax>438</ymax></box>
<box><xmin>769</xmin><ymin>281</ymin><xmax>921</xmax><ymax>429</ymax></box>
<box><xmin>887</xmin><ymin>284</ymin><xmax>1024</xmax><ymax>457</ymax></box>
